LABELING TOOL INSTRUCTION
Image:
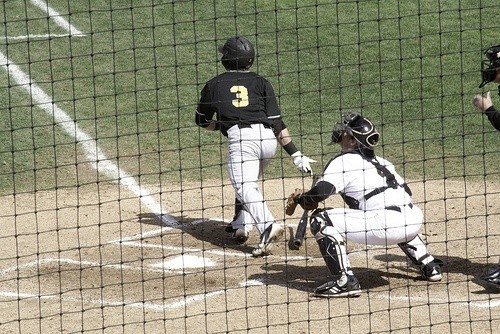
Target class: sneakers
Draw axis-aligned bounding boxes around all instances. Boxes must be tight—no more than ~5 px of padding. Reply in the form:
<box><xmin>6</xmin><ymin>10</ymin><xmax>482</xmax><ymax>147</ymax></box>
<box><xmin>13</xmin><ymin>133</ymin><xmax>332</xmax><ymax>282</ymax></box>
<box><xmin>232</xmin><ymin>229</ymin><xmax>248</xmax><ymax>242</ymax></box>
<box><xmin>316</xmin><ymin>275</ymin><xmax>361</xmax><ymax>297</ymax></box>
<box><xmin>252</xmin><ymin>224</ymin><xmax>285</xmax><ymax>256</ymax></box>
<box><xmin>421</xmin><ymin>266</ymin><xmax>442</xmax><ymax>282</ymax></box>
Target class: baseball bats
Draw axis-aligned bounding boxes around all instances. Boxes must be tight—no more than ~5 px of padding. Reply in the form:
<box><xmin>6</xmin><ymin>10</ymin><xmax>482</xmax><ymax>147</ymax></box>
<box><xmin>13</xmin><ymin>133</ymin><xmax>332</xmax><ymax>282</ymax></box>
<box><xmin>293</xmin><ymin>174</ymin><xmax>318</xmax><ymax>248</ymax></box>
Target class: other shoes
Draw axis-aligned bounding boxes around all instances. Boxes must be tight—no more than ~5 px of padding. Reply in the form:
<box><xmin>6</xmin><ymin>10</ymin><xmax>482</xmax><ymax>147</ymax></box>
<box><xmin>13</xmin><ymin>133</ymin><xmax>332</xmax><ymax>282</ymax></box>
<box><xmin>482</xmin><ymin>267</ymin><xmax>500</xmax><ymax>288</ymax></box>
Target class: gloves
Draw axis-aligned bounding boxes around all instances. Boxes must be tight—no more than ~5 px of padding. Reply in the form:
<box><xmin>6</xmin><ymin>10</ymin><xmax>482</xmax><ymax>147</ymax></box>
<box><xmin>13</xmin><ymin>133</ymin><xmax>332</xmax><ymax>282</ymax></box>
<box><xmin>291</xmin><ymin>151</ymin><xmax>316</xmax><ymax>173</ymax></box>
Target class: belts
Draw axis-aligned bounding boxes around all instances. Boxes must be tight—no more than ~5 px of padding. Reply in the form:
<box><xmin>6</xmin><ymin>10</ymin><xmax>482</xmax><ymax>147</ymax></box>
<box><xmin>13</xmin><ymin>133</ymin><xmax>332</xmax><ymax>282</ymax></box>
<box><xmin>238</xmin><ymin>122</ymin><xmax>272</xmax><ymax>129</ymax></box>
<box><xmin>384</xmin><ymin>202</ymin><xmax>413</xmax><ymax>212</ymax></box>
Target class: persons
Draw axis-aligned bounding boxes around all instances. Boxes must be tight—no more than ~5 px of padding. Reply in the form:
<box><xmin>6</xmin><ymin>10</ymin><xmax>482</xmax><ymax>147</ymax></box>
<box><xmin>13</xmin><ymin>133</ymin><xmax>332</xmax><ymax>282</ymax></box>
<box><xmin>473</xmin><ymin>44</ymin><xmax>500</xmax><ymax>288</ymax></box>
<box><xmin>195</xmin><ymin>38</ymin><xmax>317</xmax><ymax>257</ymax></box>
<box><xmin>285</xmin><ymin>113</ymin><xmax>443</xmax><ymax>297</ymax></box>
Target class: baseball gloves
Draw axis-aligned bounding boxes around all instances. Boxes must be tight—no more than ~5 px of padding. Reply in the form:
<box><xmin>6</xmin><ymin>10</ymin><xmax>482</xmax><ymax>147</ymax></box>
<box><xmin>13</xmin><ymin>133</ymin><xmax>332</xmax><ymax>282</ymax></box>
<box><xmin>285</xmin><ymin>189</ymin><xmax>318</xmax><ymax>216</ymax></box>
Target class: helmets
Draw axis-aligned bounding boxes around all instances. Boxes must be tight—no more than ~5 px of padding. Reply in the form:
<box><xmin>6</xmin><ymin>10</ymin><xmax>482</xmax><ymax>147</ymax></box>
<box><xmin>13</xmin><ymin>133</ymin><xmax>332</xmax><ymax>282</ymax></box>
<box><xmin>218</xmin><ymin>37</ymin><xmax>255</xmax><ymax>70</ymax></box>
<box><xmin>350</xmin><ymin>116</ymin><xmax>378</xmax><ymax>159</ymax></box>
